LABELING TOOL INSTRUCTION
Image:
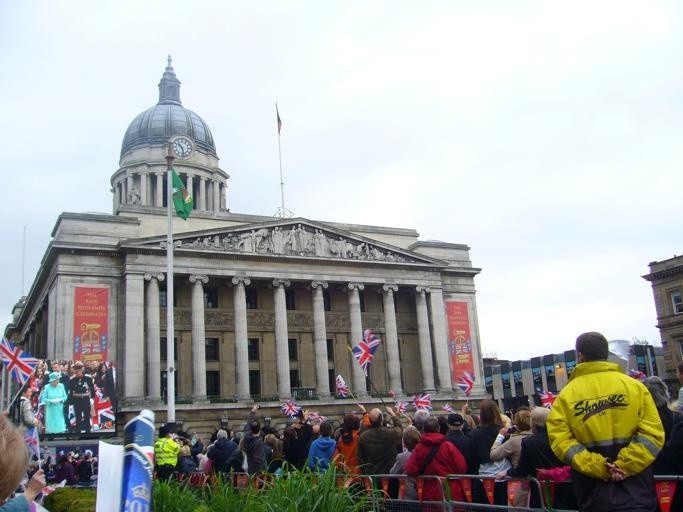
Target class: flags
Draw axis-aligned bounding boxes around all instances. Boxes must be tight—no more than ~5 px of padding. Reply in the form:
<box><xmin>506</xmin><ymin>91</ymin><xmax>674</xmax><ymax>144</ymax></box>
<box><xmin>0</xmin><ymin>336</ymin><xmax>41</xmax><ymax>385</ymax></box>
<box><xmin>172</xmin><ymin>168</ymin><xmax>193</xmax><ymax>221</ymax></box>
<box><xmin>277</xmin><ymin>109</ymin><xmax>281</xmax><ymax>134</ymax></box>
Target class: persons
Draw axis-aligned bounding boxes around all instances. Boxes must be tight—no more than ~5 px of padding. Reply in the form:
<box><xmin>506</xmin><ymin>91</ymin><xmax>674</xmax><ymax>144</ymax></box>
<box><xmin>192</xmin><ymin>223</ymin><xmax>404</xmax><ymax>261</ymax></box>
<box><xmin>0</xmin><ymin>415</ymin><xmax>98</xmax><ymax>512</ymax></box>
<box><xmin>18</xmin><ymin>357</ymin><xmax>117</xmax><ymax>433</ymax></box>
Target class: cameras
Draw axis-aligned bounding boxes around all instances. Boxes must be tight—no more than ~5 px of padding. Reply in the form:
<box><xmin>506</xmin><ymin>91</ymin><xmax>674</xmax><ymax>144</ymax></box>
<box><xmin>508</xmin><ymin>428</ymin><xmax>514</xmax><ymax>434</ymax></box>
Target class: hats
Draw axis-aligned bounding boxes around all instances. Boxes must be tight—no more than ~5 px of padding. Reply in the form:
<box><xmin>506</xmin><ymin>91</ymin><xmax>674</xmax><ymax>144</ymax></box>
<box><xmin>447</xmin><ymin>413</ymin><xmax>464</xmax><ymax>427</ymax></box>
<box><xmin>47</xmin><ymin>371</ymin><xmax>63</xmax><ymax>383</ymax></box>
<box><xmin>72</xmin><ymin>365</ymin><xmax>84</xmax><ymax>371</ymax></box>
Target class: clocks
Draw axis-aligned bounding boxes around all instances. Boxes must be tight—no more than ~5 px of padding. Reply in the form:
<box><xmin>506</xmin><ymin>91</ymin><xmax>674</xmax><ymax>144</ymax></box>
<box><xmin>171</xmin><ymin>134</ymin><xmax>193</xmax><ymax>160</ymax></box>
